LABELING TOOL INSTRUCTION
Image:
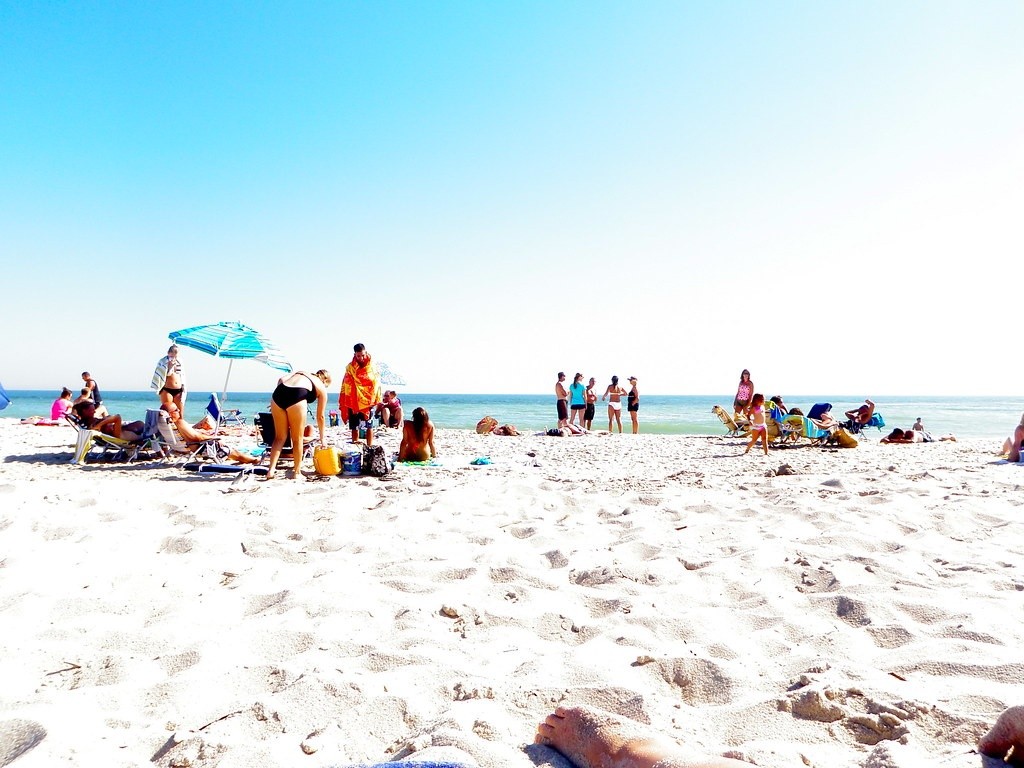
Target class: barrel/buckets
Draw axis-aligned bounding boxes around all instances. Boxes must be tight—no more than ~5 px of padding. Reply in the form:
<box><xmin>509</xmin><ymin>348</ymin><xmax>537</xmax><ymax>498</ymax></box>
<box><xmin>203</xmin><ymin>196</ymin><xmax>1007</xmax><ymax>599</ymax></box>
<box><xmin>341</xmin><ymin>452</ymin><xmax>362</xmax><ymax>475</ymax></box>
<box><xmin>313</xmin><ymin>444</ymin><xmax>342</xmax><ymax>475</ymax></box>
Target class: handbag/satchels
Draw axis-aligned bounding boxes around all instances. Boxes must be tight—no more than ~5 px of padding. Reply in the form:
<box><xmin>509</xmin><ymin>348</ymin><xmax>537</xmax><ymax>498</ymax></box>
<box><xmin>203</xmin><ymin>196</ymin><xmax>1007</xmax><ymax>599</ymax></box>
<box><xmin>362</xmin><ymin>443</ymin><xmax>388</xmax><ymax>476</ymax></box>
<box><xmin>339</xmin><ymin>450</ymin><xmax>362</xmax><ymax>476</ymax></box>
<box><xmin>314</xmin><ymin>445</ymin><xmax>341</xmax><ymax>475</ymax></box>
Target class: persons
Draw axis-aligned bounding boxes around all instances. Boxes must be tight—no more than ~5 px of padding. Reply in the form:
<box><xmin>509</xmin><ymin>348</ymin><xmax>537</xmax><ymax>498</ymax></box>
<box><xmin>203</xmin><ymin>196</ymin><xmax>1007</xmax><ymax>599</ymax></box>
<box><xmin>398</xmin><ymin>407</ymin><xmax>437</xmax><ymax>461</ymax></box>
<box><xmin>734</xmin><ymin>370</ymin><xmax>754</xmax><ymax>420</ymax></box>
<box><xmin>790</xmin><ymin>405</ymin><xmax>839</xmax><ymax>428</ymax></box>
<box><xmin>601</xmin><ymin>376</ymin><xmax>627</xmax><ymax>433</ymax></box>
<box><xmin>584</xmin><ymin>377</ymin><xmax>598</xmax><ymax>430</ymax></box>
<box><xmin>160</xmin><ymin>402</ymin><xmax>260</xmax><ymax>464</ymax></box>
<box><xmin>75</xmin><ymin>401</ymin><xmax>170</xmax><ymax>458</ymax></box>
<box><xmin>771</xmin><ymin>395</ymin><xmax>789</xmax><ymax>414</ymax></box>
<box><xmin>569</xmin><ymin>372</ymin><xmax>587</xmax><ymax>428</ymax></box>
<box><xmin>338</xmin><ymin>342</ymin><xmax>380</xmax><ymax>447</ymax></box>
<box><xmin>846</xmin><ymin>399</ymin><xmax>875</xmax><ymax>425</ymax></box>
<box><xmin>267</xmin><ymin>369</ymin><xmax>332</xmax><ymax>480</ymax></box>
<box><xmin>537</xmin><ymin>703</ymin><xmax>1024</xmax><ymax>768</ymax></box>
<box><xmin>626</xmin><ymin>377</ymin><xmax>640</xmax><ymax>435</ymax></box>
<box><xmin>1002</xmin><ymin>414</ymin><xmax>1024</xmax><ymax>462</ymax></box>
<box><xmin>375</xmin><ymin>390</ymin><xmax>402</xmax><ymax>427</ymax></box>
<box><xmin>149</xmin><ymin>344</ymin><xmax>188</xmax><ymax>419</ymax></box>
<box><xmin>744</xmin><ymin>394</ymin><xmax>769</xmax><ymax>455</ymax></box>
<box><xmin>555</xmin><ymin>371</ymin><xmax>569</xmax><ymax>429</ymax></box>
<box><xmin>51</xmin><ymin>371</ymin><xmax>110</xmax><ymax>420</ymax></box>
<box><xmin>882</xmin><ymin>417</ymin><xmax>956</xmax><ymax>444</ymax></box>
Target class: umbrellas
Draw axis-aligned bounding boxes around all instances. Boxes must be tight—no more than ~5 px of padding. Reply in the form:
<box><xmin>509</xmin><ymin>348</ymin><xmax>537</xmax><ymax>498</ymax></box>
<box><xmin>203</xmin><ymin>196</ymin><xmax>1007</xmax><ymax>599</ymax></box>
<box><xmin>167</xmin><ymin>318</ymin><xmax>294</xmax><ymax>437</ymax></box>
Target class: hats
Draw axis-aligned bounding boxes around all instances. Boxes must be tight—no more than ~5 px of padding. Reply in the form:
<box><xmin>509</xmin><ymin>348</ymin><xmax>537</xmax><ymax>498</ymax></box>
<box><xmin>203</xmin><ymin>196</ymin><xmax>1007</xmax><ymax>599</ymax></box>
<box><xmin>627</xmin><ymin>376</ymin><xmax>636</xmax><ymax>380</ymax></box>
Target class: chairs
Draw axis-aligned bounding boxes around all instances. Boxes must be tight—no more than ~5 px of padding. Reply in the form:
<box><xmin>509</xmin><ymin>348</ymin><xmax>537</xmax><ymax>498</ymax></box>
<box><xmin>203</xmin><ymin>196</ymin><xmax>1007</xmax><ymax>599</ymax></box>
<box><xmin>713</xmin><ymin>405</ymin><xmax>881</xmax><ymax>448</ymax></box>
<box><xmin>62</xmin><ymin>412</ymin><xmax>166</xmax><ymax>465</ymax></box>
<box><xmin>210</xmin><ymin>393</ymin><xmax>247</xmax><ymax>435</ymax></box>
<box><xmin>255</xmin><ymin>412</ymin><xmax>322</xmax><ymax>477</ymax></box>
<box><xmin>145</xmin><ymin>409</ymin><xmax>221</xmax><ymax>469</ymax></box>
